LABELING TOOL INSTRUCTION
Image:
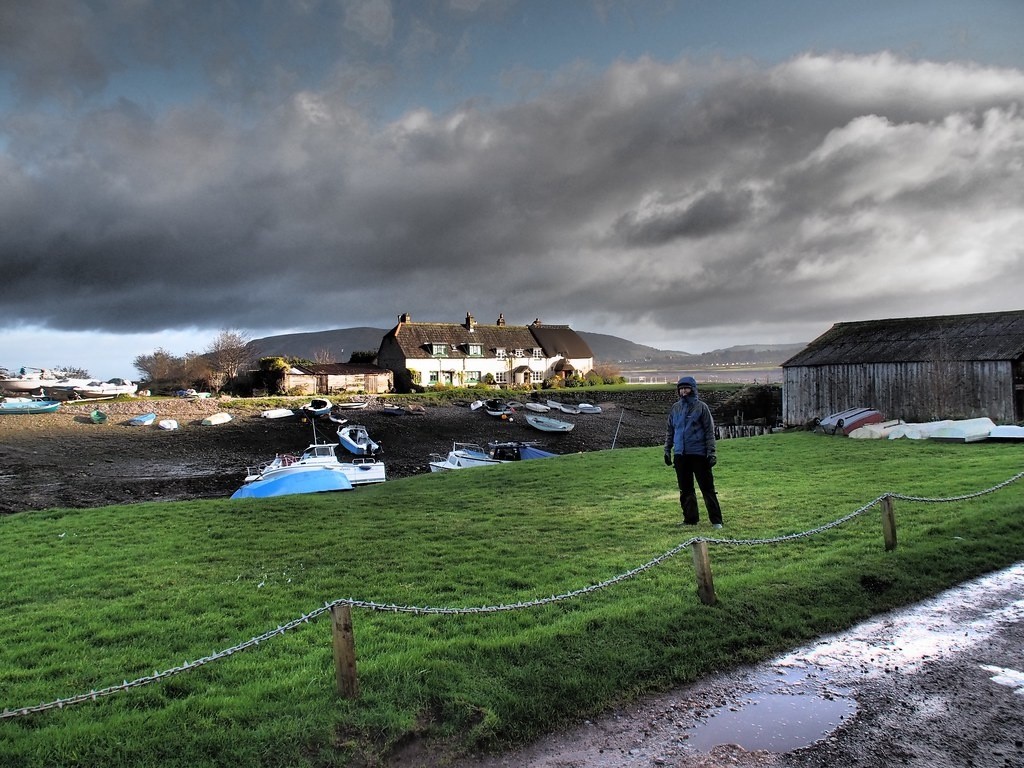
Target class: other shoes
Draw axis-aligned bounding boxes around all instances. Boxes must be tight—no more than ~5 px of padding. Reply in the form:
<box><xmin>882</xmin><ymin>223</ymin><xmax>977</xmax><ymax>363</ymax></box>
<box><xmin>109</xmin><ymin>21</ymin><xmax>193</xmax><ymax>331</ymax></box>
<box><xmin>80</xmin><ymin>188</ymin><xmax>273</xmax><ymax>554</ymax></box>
<box><xmin>713</xmin><ymin>523</ymin><xmax>723</xmax><ymax>529</ymax></box>
<box><xmin>677</xmin><ymin>520</ymin><xmax>697</xmax><ymax>526</ymax></box>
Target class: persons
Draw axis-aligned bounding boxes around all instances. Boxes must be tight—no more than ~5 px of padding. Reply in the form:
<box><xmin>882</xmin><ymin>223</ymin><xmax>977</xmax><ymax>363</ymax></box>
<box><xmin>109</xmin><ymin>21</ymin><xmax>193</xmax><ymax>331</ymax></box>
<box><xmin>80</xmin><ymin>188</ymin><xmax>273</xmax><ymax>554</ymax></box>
<box><xmin>664</xmin><ymin>377</ymin><xmax>723</xmax><ymax>529</ymax></box>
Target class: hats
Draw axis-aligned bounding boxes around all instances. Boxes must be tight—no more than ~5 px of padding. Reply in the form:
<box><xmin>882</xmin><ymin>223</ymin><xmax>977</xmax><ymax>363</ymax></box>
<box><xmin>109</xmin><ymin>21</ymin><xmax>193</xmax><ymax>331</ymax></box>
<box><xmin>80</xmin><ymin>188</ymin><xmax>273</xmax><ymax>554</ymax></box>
<box><xmin>678</xmin><ymin>383</ymin><xmax>691</xmax><ymax>389</ymax></box>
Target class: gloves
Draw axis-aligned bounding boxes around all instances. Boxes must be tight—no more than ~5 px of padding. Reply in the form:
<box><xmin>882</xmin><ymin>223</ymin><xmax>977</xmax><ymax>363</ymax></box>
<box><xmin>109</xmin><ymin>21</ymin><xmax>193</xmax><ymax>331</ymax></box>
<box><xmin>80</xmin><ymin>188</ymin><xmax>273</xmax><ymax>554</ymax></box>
<box><xmin>664</xmin><ymin>454</ymin><xmax>672</xmax><ymax>466</ymax></box>
<box><xmin>708</xmin><ymin>456</ymin><xmax>716</xmax><ymax>467</ymax></box>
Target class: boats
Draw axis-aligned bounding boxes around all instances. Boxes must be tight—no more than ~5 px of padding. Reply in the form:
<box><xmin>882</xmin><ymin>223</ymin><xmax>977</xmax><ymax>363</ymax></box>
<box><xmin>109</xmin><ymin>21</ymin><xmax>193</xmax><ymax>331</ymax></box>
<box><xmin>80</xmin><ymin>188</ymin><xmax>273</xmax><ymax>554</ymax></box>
<box><xmin>547</xmin><ymin>400</ymin><xmax>581</xmax><ymax>414</ymax></box>
<box><xmin>336</xmin><ymin>425</ymin><xmax>379</xmax><ymax>455</ymax></box>
<box><xmin>409</xmin><ymin>404</ymin><xmax>426</xmax><ymax>415</ymax></box>
<box><xmin>129</xmin><ymin>413</ymin><xmax>156</xmax><ymax>426</ymax></box>
<box><xmin>260</xmin><ymin>418</ymin><xmax>386</xmax><ymax>485</ymax></box>
<box><xmin>482</xmin><ymin>398</ymin><xmax>517</xmax><ymax>416</ymax></box>
<box><xmin>228</xmin><ymin>462</ymin><xmax>355</xmax><ymax>499</ymax></box>
<box><xmin>579</xmin><ymin>403</ymin><xmax>601</xmax><ymax>413</ymax></box>
<box><xmin>384</xmin><ymin>403</ymin><xmax>405</xmax><ymax>415</ymax></box>
<box><xmin>202</xmin><ymin>412</ymin><xmax>231</xmax><ymax>426</ymax></box>
<box><xmin>337</xmin><ymin>399</ymin><xmax>370</xmax><ymax>409</ymax></box>
<box><xmin>159</xmin><ymin>419</ymin><xmax>178</xmax><ymax>430</ymax></box>
<box><xmin>0</xmin><ymin>369</ymin><xmax>137</xmax><ymax>398</ymax></box>
<box><xmin>507</xmin><ymin>401</ymin><xmax>523</xmax><ymax>408</ymax></box>
<box><xmin>187</xmin><ymin>392</ymin><xmax>211</xmax><ymax>398</ymax></box>
<box><xmin>0</xmin><ymin>402</ymin><xmax>62</xmax><ymax>414</ymax></box>
<box><xmin>429</xmin><ymin>442</ymin><xmax>559</xmax><ymax>472</ymax></box>
<box><xmin>91</xmin><ymin>409</ymin><xmax>108</xmax><ymax>423</ymax></box>
<box><xmin>525</xmin><ymin>414</ymin><xmax>575</xmax><ymax>431</ymax></box>
<box><xmin>299</xmin><ymin>398</ymin><xmax>332</xmax><ymax>418</ymax></box>
<box><xmin>471</xmin><ymin>400</ymin><xmax>482</xmax><ymax>410</ymax></box>
<box><xmin>526</xmin><ymin>403</ymin><xmax>550</xmax><ymax>412</ymax></box>
<box><xmin>261</xmin><ymin>409</ymin><xmax>294</xmax><ymax>419</ymax></box>
<box><xmin>328</xmin><ymin>411</ymin><xmax>348</xmax><ymax>423</ymax></box>
